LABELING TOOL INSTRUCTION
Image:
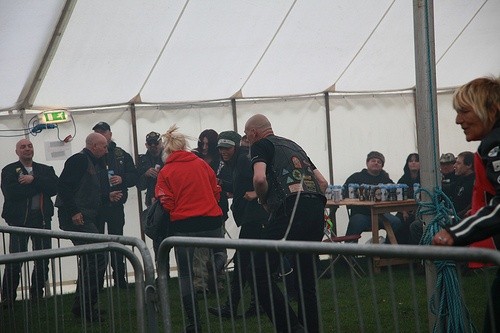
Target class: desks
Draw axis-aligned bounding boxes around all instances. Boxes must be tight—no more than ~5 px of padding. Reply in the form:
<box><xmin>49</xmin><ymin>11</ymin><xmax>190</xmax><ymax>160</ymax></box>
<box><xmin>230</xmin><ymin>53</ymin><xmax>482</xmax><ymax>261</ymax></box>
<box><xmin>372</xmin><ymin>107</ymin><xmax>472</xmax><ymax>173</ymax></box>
<box><xmin>324</xmin><ymin>198</ymin><xmax>421</xmax><ymax>274</ymax></box>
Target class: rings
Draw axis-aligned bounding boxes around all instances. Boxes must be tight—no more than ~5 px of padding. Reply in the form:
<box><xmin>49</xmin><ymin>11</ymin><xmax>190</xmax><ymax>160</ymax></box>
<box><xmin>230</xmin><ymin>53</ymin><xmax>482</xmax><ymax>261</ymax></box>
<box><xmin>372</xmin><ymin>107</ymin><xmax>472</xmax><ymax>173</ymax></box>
<box><xmin>438</xmin><ymin>237</ymin><xmax>445</xmax><ymax>241</ymax></box>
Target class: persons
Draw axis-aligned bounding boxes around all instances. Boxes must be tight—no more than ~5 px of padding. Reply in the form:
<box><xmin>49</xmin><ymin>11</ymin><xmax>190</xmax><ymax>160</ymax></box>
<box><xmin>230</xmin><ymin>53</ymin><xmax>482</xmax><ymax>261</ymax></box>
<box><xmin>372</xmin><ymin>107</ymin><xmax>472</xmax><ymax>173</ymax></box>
<box><xmin>136</xmin><ymin>131</ymin><xmax>173</xmax><ymax>285</ymax></box>
<box><xmin>385</xmin><ymin>153</ymin><xmax>420</xmax><ymax>273</ymax></box>
<box><xmin>341</xmin><ymin>151</ymin><xmax>404</xmax><ymax>274</ymax></box>
<box><xmin>244</xmin><ymin>114</ymin><xmax>328</xmax><ymax>333</ymax></box>
<box><xmin>208</xmin><ymin>130</ymin><xmax>270</xmax><ymax>319</ymax></box>
<box><xmin>92</xmin><ymin>122</ymin><xmax>138</xmax><ymax>295</ymax></box>
<box><xmin>53</xmin><ymin>132</ymin><xmax>124</xmax><ymax>323</ymax></box>
<box><xmin>0</xmin><ymin>139</ymin><xmax>59</xmax><ymax>323</ymax></box>
<box><xmin>447</xmin><ymin>151</ymin><xmax>475</xmax><ymax>221</ymax></box>
<box><xmin>409</xmin><ymin>152</ymin><xmax>456</xmax><ymax>245</ymax></box>
<box><xmin>430</xmin><ymin>77</ymin><xmax>500</xmax><ymax>333</ymax></box>
<box><xmin>190</xmin><ymin>129</ymin><xmax>220</xmax><ymax>176</ymax></box>
<box><xmin>152</xmin><ymin>124</ymin><xmax>228</xmax><ymax>333</ymax></box>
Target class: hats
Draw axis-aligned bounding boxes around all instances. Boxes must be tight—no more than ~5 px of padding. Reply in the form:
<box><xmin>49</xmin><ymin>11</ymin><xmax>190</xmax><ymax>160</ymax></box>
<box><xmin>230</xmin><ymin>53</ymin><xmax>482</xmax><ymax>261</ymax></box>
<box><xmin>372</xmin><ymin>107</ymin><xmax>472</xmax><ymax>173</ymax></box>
<box><xmin>216</xmin><ymin>131</ymin><xmax>241</xmax><ymax>147</ymax></box>
<box><xmin>146</xmin><ymin>132</ymin><xmax>161</xmax><ymax>141</ymax></box>
<box><xmin>439</xmin><ymin>152</ymin><xmax>455</xmax><ymax>163</ymax></box>
<box><xmin>92</xmin><ymin>121</ymin><xmax>111</xmax><ymax>131</ymax></box>
<box><xmin>367</xmin><ymin>151</ymin><xmax>386</xmax><ymax>168</ymax></box>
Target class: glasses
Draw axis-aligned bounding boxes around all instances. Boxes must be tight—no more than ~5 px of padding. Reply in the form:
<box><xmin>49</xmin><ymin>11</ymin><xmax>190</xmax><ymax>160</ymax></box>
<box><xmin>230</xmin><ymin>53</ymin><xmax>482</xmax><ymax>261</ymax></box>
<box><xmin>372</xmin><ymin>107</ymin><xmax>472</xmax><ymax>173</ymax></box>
<box><xmin>147</xmin><ymin>140</ymin><xmax>161</xmax><ymax>146</ymax></box>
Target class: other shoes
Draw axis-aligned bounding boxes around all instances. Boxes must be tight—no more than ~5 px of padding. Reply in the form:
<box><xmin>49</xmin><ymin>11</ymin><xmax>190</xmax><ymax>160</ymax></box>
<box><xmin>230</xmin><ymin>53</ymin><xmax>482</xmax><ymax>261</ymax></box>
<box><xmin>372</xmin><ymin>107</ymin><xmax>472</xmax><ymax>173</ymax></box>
<box><xmin>208</xmin><ymin>304</ymin><xmax>237</xmax><ymax>318</ymax></box>
<box><xmin>1</xmin><ymin>294</ymin><xmax>17</xmax><ymax>307</ymax></box>
<box><xmin>114</xmin><ymin>280</ymin><xmax>132</xmax><ymax>288</ymax></box>
<box><xmin>206</xmin><ymin>261</ymin><xmax>223</xmax><ymax>292</ymax></box>
<box><xmin>72</xmin><ymin>308</ymin><xmax>104</xmax><ymax>322</ymax></box>
<box><xmin>30</xmin><ymin>295</ymin><xmax>47</xmax><ymax>304</ymax></box>
<box><xmin>238</xmin><ymin>307</ymin><xmax>265</xmax><ymax>317</ymax></box>
<box><xmin>195</xmin><ymin>287</ymin><xmax>210</xmax><ymax>294</ymax></box>
<box><xmin>92</xmin><ymin>309</ymin><xmax>107</xmax><ymax>315</ymax></box>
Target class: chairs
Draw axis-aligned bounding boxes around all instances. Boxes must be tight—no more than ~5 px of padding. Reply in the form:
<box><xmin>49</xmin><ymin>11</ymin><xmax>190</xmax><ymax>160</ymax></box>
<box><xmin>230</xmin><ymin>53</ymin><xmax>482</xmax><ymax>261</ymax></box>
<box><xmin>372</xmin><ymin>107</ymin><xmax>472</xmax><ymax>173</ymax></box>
<box><xmin>316</xmin><ymin>209</ymin><xmax>368</xmax><ymax>279</ymax></box>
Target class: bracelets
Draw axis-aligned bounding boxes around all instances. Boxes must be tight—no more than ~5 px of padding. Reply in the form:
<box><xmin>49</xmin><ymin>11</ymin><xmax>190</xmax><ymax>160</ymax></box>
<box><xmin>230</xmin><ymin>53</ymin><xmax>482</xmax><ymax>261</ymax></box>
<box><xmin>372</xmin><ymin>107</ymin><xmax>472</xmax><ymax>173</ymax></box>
<box><xmin>257</xmin><ymin>197</ymin><xmax>262</xmax><ymax>205</ymax></box>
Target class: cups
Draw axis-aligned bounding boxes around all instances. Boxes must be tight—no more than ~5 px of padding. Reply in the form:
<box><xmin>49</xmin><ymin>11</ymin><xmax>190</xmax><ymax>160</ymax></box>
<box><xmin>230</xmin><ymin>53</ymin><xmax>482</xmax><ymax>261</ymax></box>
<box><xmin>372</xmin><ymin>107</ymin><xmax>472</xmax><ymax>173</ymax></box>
<box><xmin>154</xmin><ymin>164</ymin><xmax>160</xmax><ymax>171</ymax></box>
<box><xmin>326</xmin><ymin>183</ymin><xmax>420</xmax><ymax>203</ymax></box>
<box><xmin>108</xmin><ymin>170</ymin><xmax>115</xmax><ymax>187</ymax></box>
